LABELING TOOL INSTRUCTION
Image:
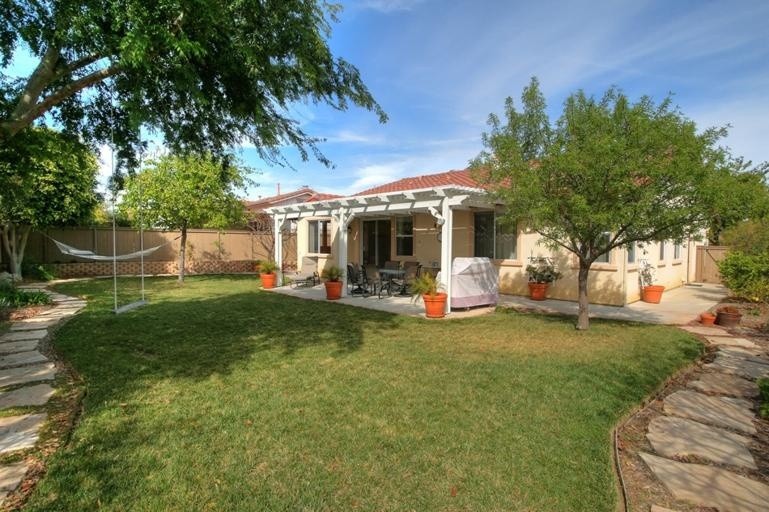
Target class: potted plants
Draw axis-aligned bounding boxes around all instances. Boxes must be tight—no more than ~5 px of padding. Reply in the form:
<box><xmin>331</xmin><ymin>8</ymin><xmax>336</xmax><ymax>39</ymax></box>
<box><xmin>637</xmin><ymin>258</ymin><xmax>664</xmax><ymax>304</ymax></box>
<box><xmin>522</xmin><ymin>256</ymin><xmax>563</xmax><ymax>301</ymax></box>
<box><xmin>257</xmin><ymin>258</ymin><xmax>279</xmax><ymax>288</ymax></box>
<box><xmin>406</xmin><ymin>274</ymin><xmax>448</xmax><ymax>317</ymax></box>
<box><xmin>323</xmin><ymin>266</ymin><xmax>345</xmax><ymax>300</ymax></box>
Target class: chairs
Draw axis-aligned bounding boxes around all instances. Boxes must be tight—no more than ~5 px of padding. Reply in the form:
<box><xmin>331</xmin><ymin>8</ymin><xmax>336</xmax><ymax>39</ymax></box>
<box><xmin>348</xmin><ymin>261</ymin><xmax>422</xmax><ymax>299</ymax></box>
<box><xmin>294</xmin><ymin>256</ymin><xmax>320</xmax><ymax>287</ymax></box>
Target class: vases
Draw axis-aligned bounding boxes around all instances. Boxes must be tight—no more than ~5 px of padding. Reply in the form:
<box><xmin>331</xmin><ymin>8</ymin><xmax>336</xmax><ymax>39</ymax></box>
<box><xmin>700</xmin><ymin>307</ymin><xmax>741</xmax><ymax>326</ymax></box>
